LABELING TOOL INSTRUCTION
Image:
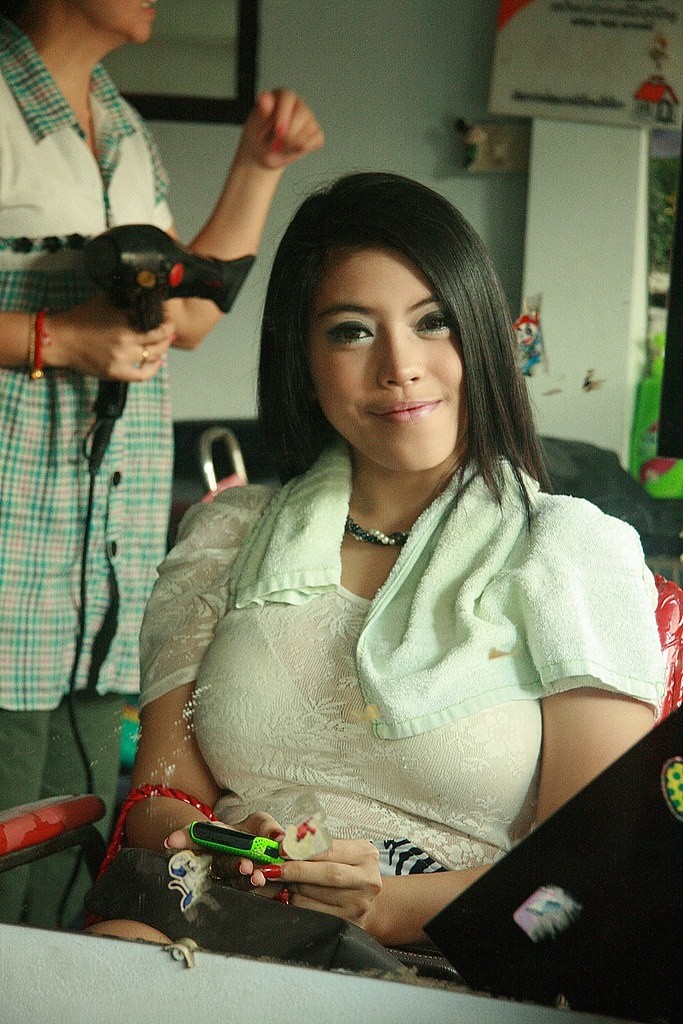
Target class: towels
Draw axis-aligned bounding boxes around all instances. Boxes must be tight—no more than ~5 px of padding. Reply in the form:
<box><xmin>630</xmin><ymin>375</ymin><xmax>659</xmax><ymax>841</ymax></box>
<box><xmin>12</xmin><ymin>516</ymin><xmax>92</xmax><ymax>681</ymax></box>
<box><xmin>227</xmin><ymin>436</ymin><xmax>662</xmax><ymax>740</ymax></box>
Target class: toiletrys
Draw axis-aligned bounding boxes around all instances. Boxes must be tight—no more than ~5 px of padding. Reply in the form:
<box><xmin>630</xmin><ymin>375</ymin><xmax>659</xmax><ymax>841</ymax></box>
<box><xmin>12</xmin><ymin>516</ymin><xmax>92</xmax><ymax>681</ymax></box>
<box><xmin>630</xmin><ymin>333</ymin><xmax>682</xmax><ymax>500</ymax></box>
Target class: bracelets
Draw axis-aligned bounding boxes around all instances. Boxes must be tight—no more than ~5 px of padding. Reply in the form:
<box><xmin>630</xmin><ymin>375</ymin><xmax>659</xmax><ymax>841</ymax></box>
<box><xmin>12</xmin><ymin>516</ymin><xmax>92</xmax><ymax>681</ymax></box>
<box><xmin>29</xmin><ymin>311</ymin><xmax>45</xmax><ymax>379</ymax></box>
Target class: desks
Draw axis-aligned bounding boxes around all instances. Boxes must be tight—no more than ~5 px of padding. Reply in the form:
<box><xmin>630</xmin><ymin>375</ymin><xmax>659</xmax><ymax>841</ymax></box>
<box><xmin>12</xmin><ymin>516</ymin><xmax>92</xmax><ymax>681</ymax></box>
<box><xmin>0</xmin><ymin>923</ymin><xmax>641</xmax><ymax>1024</ymax></box>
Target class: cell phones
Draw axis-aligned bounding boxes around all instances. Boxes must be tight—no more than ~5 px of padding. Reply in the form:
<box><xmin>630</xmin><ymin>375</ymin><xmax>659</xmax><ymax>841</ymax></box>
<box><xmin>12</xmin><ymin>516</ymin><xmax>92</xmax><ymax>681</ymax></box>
<box><xmin>188</xmin><ymin>820</ymin><xmax>285</xmax><ymax>865</ymax></box>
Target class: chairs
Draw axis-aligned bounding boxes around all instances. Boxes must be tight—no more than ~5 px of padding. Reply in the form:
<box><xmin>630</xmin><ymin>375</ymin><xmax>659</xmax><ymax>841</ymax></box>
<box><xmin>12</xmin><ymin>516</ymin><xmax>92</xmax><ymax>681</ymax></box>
<box><xmin>0</xmin><ymin>571</ymin><xmax>683</xmax><ymax>880</ymax></box>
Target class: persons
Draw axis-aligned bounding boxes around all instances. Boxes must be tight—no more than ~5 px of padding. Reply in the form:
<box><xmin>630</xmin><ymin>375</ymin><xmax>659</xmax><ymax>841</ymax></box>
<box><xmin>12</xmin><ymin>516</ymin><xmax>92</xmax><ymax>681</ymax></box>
<box><xmin>0</xmin><ymin>4</ymin><xmax>325</xmax><ymax>930</ymax></box>
<box><xmin>82</xmin><ymin>172</ymin><xmax>667</xmax><ymax>945</ymax></box>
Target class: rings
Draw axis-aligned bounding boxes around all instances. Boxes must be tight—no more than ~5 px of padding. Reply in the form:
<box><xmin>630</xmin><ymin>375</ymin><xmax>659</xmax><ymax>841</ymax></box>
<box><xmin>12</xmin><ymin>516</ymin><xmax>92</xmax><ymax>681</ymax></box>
<box><xmin>141</xmin><ymin>345</ymin><xmax>149</xmax><ymax>364</ymax></box>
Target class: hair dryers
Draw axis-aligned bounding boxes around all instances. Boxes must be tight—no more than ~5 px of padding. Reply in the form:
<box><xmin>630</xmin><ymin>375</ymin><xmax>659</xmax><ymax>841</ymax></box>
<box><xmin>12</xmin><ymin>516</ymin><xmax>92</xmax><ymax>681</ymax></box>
<box><xmin>85</xmin><ymin>224</ymin><xmax>255</xmax><ymax>481</ymax></box>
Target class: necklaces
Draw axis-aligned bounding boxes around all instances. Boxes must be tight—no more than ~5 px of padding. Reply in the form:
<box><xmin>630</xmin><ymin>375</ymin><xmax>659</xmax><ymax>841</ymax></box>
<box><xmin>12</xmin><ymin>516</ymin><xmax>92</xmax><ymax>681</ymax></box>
<box><xmin>345</xmin><ymin>515</ymin><xmax>410</xmax><ymax>546</ymax></box>
